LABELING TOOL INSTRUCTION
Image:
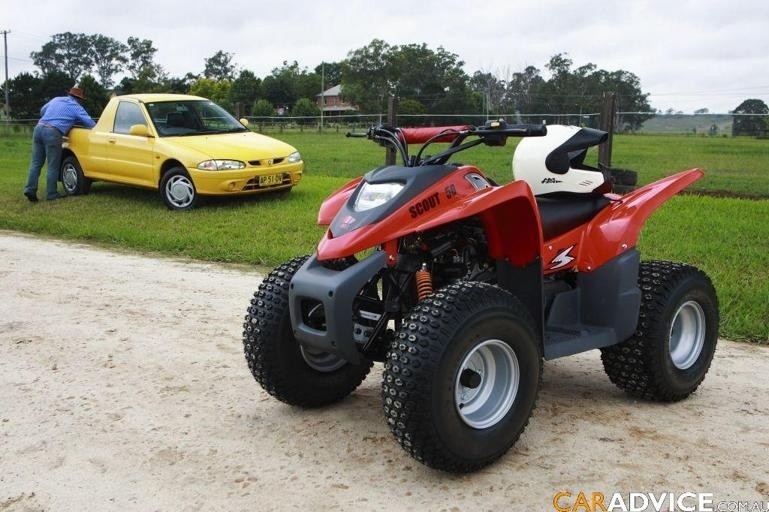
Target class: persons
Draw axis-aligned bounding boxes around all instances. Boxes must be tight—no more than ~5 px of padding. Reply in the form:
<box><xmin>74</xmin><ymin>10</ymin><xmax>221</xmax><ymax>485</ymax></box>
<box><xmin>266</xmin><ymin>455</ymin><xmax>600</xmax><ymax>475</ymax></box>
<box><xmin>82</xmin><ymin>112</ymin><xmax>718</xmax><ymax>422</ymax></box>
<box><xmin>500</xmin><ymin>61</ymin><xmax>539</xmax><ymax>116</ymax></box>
<box><xmin>23</xmin><ymin>86</ymin><xmax>97</xmax><ymax>203</ymax></box>
<box><xmin>710</xmin><ymin>125</ymin><xmax>716</xmax><ymax>134</ymax></box>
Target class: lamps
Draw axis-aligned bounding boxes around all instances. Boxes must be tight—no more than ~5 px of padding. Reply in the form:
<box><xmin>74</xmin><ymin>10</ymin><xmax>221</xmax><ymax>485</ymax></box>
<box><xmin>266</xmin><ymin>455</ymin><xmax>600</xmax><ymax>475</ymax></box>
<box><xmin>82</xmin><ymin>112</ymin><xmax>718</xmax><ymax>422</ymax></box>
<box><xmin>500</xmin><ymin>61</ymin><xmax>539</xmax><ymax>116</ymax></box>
<box><xmin>166</xmin><ymin>111</ymin><xmax>195</xmax><ymax>128</ymax></box>
<box><xmin>513</xmin><ymin>125</ymin><xmax>613</xmax><ymax>240</ymax></box>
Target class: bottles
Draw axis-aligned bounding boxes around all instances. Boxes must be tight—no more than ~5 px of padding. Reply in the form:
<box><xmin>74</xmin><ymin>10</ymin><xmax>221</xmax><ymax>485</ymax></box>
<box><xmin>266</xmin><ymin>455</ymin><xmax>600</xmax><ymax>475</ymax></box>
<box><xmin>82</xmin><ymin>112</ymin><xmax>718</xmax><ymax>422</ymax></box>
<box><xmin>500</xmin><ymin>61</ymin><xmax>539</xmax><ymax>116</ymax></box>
<box><xmin>67</xmin><ymin>88</ymin><xmax>83</xmax><ymax>99</ymax></box>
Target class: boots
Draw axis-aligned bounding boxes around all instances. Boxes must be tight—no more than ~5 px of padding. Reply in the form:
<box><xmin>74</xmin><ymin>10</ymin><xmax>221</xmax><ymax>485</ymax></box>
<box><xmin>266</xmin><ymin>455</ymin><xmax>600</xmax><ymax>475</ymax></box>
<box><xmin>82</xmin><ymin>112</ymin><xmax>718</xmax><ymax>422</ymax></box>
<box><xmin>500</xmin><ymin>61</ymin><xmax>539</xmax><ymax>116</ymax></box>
<box><xmin>242</xmin><ymin>112</ymin><xmax>721</xmax><ymax>474</ymax></box>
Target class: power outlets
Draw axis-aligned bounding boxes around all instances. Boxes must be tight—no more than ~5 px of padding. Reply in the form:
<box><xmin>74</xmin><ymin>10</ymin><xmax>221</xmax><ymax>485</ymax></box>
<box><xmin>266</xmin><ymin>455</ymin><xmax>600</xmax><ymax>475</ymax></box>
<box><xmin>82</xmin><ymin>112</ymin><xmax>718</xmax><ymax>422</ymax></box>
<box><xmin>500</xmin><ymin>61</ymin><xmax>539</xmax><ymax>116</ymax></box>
<box><xmin>56</xmin><ymin>92</ymin><xmax>303</xmax><ymax>210</ymax></box>
